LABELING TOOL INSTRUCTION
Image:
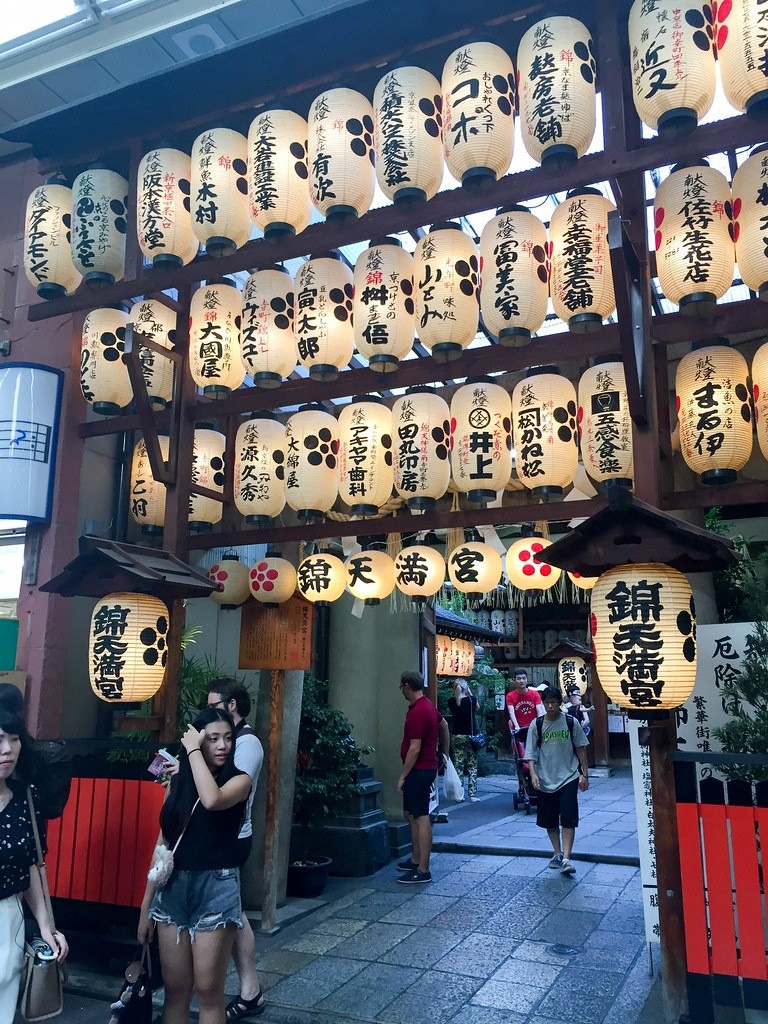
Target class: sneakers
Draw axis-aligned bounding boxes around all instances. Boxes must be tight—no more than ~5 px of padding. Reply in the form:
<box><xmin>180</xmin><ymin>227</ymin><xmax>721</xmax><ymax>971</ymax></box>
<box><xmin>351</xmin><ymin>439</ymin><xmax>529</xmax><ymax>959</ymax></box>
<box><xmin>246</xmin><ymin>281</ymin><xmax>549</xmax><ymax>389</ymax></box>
<box><xmin>397</xmin><ymin>858</ymin><xmax>419</xmax><ymax>870</ymax></box>
<box><xmin>559</xmin><ymin>861</ymin><xmax>576</xmax><ymax>873</ymax></box>
<box><xmin>549</xmin><ymin>852</ymin><xmax>563</xmax><ymax>867</ymax></box>
<box><xmin>397</xmin><ymin>870</ymin><xmax>431</xmax><ymax>883</ymax></box>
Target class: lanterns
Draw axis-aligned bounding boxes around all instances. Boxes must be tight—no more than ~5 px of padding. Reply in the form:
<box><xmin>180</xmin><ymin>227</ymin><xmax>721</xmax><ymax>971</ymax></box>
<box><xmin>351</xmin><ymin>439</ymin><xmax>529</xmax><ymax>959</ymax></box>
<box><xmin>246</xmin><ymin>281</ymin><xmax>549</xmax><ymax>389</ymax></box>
<box><xmin>555</xmin><ymin>656</ymin><xmax>589</xmax><ymax>700</ymax></box>
<box><xmin>87</xmin><ymin>591</ymin><xmax>171</xmax><ymax>714</ymax></box>
<box><xmin>435</xmin><ymin>632</ymin><xmax>477</xmax><ymax>677</ymax></box>
<box><xmin>589</xmin><ymin>557</ymin><xmax>697</xmax><ymax>724</ymax></box>
<box><xmin>18</xmin><ymin>0</ymin><xmax>768</xmax><ymax>600</ymax></box>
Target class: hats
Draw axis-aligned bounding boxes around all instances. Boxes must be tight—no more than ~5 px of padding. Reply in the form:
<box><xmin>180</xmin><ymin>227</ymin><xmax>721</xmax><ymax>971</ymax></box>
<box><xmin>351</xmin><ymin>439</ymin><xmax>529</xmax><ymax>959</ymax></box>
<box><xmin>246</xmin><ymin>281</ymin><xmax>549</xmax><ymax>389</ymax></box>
<box><xmin>528</xmin><ymin>684</ymin><xmax>547</xmax><ymax>691</ymax></box>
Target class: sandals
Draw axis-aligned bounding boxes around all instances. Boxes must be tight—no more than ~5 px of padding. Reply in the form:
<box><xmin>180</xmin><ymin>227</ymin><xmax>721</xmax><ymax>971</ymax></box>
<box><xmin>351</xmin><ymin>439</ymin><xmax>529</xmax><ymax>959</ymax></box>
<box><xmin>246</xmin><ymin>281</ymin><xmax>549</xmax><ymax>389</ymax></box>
<box><xmin>225</xmin><ymin>991</ymin><xmax>265</xmax><ymax>1021</ymax></box>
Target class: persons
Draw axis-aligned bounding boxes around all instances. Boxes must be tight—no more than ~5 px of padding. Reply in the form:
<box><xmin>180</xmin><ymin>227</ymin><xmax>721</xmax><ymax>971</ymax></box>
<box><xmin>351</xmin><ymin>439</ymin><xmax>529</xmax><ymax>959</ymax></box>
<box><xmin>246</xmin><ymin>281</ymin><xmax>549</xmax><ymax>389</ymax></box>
<box><xmin>154</xmin><ymin>678</ymin><xmax>267</xmax><ymax>1019</ymax></box>
<box><xmin>525</xmin><ymin>680</ymin><xmax>596</xmax><ymax>737</ymax></box>
<box><xmin>523</xmin><ymin>687</ymin><xmax>591</xmax><ymax>873</ymax></box>
<box><xmin>0</xmin><ymin>682</ymin><xmax>69</xmax><ymax>1023</ymax></box>
<box><xmin>506</xmin><ymin>669</ymin><xmax>542</xmax><ymax>732</ymax></box>
<box><xmin>137</xmin><ymin>707</ymin><xmax>255</xmax><ymax>1024</ymax></box>
<box><xmin>396</xmin><ymin>670</ymin><xmax>450</xmax><ymax>885</ymax></box>
<box><xmin>447</xmin><ymin>678</ymin><xmax>481</xmax><ymax>802</ymax></box>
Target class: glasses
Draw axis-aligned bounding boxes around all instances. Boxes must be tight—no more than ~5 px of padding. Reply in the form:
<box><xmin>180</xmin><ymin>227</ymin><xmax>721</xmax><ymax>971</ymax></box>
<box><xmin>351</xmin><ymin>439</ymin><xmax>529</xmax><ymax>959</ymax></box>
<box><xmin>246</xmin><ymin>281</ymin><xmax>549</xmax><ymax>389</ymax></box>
<box><xmin>399</xmin><ymin>684</ymin><xmax>405</xmax><ymax>689</ymax></box>
<box><xmin>206</xmin><ymin>701</ymin><xmax>224</xmax><ymax>708</ymax></box>
<box><xmin>543</xmin><ymin>700</ymin><xmax>559</xmax><ymax>706</ymax></box>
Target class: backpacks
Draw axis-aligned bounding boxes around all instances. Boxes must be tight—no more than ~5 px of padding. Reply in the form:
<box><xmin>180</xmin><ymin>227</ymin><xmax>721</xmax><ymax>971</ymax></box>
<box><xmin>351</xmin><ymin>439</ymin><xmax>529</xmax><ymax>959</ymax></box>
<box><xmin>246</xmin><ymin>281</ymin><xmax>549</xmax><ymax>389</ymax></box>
<box><xmin>34</xmin><ymin>740</ymin><xmax>70</xmax><ymax>819</ymax></box>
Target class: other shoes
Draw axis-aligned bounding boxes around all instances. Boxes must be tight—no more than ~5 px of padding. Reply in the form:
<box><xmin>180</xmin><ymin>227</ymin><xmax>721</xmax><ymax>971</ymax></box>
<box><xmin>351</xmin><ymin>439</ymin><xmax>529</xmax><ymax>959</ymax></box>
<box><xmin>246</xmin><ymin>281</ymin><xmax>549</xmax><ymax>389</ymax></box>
<box><xmin>470</xmin><ymin>796</ymin><xmax>481</xmax><ymax>803</ymax></box>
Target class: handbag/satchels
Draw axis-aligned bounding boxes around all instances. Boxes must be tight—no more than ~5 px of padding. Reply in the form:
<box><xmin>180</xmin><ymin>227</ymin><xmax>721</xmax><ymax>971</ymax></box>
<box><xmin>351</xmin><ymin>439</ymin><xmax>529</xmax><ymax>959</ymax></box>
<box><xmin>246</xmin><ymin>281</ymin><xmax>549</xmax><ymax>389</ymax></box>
<box><xmin>470</xmin><ymin>732</ymin><xmax>486</xmax><ymax>750</ymax></box>
<box><xmin>108</xmin><ymin>941</ymin><xmax>152</xmax><ymax>1024</ymax></box>
<box><xmin>443</xmin><ymin>753</ymin><xmax>464</xmax><ymax>801</ymax></box>
<box><xmin>19</xmin><ymin>937</ymin><xmax>63</xmax><ymax>1022</ymax></box>
<box><xmin>147</xmin><ymin>845</ymin><xmax>174</xmax><ymax>887</ymax></box>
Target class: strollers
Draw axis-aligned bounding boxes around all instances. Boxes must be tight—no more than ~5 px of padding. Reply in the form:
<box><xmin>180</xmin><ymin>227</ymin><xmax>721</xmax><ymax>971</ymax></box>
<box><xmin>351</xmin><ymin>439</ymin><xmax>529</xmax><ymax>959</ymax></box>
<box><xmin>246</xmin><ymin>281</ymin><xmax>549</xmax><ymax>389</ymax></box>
<box><xmin>510</xmin><ymin>725</ymin><xmax>538</xmax><ymax>815</ymax></box>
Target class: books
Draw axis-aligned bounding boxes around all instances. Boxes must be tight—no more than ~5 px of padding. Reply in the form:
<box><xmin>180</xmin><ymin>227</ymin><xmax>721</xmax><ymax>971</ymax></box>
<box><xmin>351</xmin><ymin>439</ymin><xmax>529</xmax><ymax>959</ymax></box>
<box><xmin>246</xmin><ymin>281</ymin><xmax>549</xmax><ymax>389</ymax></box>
<box><xmin>147</xmin><ymin>750</ymin><xmax>180</xmax><ymax>784</ymax></box>
